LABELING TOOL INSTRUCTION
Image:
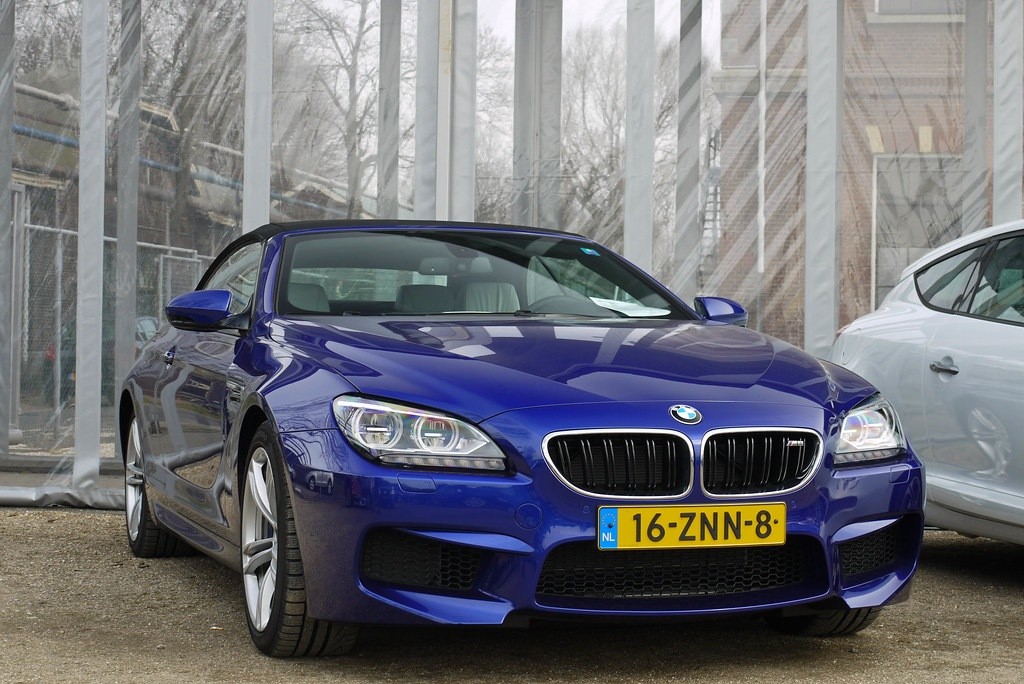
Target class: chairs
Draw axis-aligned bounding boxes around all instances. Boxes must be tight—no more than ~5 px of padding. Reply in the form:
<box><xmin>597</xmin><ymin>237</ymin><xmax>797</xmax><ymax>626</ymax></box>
<box><xmin>461</xmin><ymin>282</ymin><xmax>520</xmax><ymax>312</ymax></box>
<box><xmin>289</xmin><ymin>283</ymin><xmax>330</xmax><ymax>312</ymax></box>
<box><xmin>395</xmin><ymin>284</ymin><xmax>456</xmax><ymax>311</ymax></box>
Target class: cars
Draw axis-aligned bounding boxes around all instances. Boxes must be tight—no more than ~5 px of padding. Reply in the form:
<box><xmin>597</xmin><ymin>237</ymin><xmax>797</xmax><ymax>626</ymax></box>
<box><xmin>119</xmin><ymin>220</ymin><xmax>926</xmax><ymax>653</ymax></box>
<box><xmin>223</xmin><ymin>364</ymin><xmax>264</xmax><ymax>437</ymax></box>
<box><xmin>447</xmin><ymin>481</ymin><xmax>554</xmax><ymax>533</ymax></box>
<box><xmin>46</xmin><ymin>312</ymin><xmax>159</xmax><ymax>404</ymax></box>
<box><xmin>345</xmin><ymin>476</ymin><xmax>380</xmax><ymax>507</ymax></box>
<box><xmin>817</xmin><ymin>219</ymin><xmax>1024</xmax><ymax>547</ymax></box>
<box><xmin>303</xmin><ymin>468</ymin><xmax>334</xmax><ymax>494</ymax></box>
<box><xmin>287</xmin><ymin>462</ymin><xmax>296</xmax><ymax>480</ymax></box>
<box><xmin>377</xmin><ymin>484</ymin><xmax>398</xmax><ymax>509</ymax></box>
